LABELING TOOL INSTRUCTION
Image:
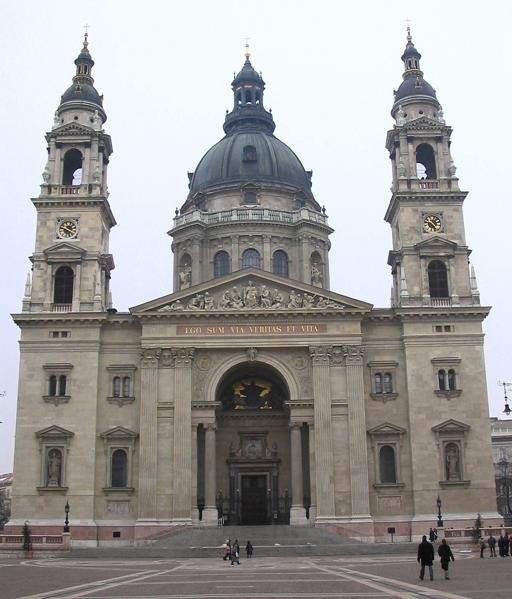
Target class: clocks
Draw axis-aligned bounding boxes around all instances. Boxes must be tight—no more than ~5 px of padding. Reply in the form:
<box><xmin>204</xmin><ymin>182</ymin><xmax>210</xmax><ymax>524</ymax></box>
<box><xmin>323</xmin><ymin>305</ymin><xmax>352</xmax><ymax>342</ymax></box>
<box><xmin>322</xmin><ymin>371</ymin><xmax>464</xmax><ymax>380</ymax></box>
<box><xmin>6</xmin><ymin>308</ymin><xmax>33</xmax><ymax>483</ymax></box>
<box><xmin>424</xmin><ymin>214</ymin><xmax>442</xmax><ymax>233</ymax></box>
<box><xmin>57</xmin><ymin>219</ymin><xmax>78</xmax><ymax>238</ymax></box>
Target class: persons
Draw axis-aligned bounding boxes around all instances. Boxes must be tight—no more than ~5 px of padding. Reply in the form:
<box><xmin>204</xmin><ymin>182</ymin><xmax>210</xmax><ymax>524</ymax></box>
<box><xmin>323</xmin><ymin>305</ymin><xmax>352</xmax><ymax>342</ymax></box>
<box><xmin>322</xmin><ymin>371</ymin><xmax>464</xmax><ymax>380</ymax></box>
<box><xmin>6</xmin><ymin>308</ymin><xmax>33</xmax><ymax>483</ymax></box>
<box><xmin>416</xmin><ymin>534</ymin><xmax>434</xmax><ymax>581</ymax></box>
<box><xmin>244</xmin><ymin>540</ymin><xmax>253</xmax><ymax>558</ymax></box>
<box><xmin>433</xmin><ymin>530</ymin><xmax>437</xmax><ymax>540</ymax></box>
<box><xmin>498</xmin><ymin>535</ymin><xmax>505</xmax><ymax>558</ymax></box>
<box><xmin>438</xmin><ymin>538</ymin><xmax>455</xmax><ymax>581</ymax></box>
<box><xmin>488</xmin><ymin>534</ymin><xmax>497</xmax><ymax>557</ymax></box>
<box><xmin>503</xmin><ymin>531</ymin><xmax>509</xmax><ymax>556</ymax></box>
<box><xmin>429</xmin><ymin>528</ymin><xmax>434</xmax><ymax>543</ymax></box>
<box><xmin>48</xmin><ymin>451</ymin><xmax>61</xmax><ymax>488</ymax></box>
<box><xmin>479</xmin><ymin>537</ymin><xmax>484</xmax><ymax>559</ymax></box>
<box><xmin>231</xmin><ymin>540</ymin><xmax>241</xmax><ymax>565</ymax></box>
<box><xmin>432</xmin><ymin>527</ymin><xmax>437</xmax><ymax>535</ymax></box>
<box><xmin>445</xmin><ymin>445</ymin><xmax>459</xmax><ymax>480</ymax></box>
<box><xmin>216</xmin><ymin>488</ymin><xmax>225</xmax><ymax>520</ymax></box>
<box><xmin>222</xmin><ymin>540</ymin><xmax>232</xmax><ymax>561</ymax></box>
<box><xmin>157</xmin><ymin>280</ymin><xmax>345</xmax><ymax>311</ymax></box>
<box><xmin>508</xmin><ymin>536</ymin><xmax>512</xmax><ymax>556</ymax></box>
